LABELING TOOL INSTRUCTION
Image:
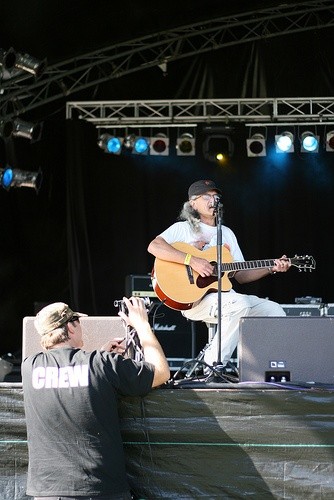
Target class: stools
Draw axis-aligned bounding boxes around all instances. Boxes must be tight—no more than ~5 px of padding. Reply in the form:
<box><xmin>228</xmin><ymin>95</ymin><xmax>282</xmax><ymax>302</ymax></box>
<box><xmin>169</xmin><ymin>318</ymin><xmax>235</xmax><ymax>386</ymax></box>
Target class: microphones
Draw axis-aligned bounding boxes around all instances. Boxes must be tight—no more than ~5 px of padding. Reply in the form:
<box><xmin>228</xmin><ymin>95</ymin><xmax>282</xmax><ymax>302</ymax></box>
<box><xmin>212</xmin><ymin>195</ymin><xmax>220</xmax><ymax>207</ymax></box>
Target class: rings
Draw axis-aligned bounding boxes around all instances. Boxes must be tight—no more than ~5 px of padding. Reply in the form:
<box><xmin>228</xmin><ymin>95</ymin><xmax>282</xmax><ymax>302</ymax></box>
<box><xmin>280</xmin><ymin>265</ymin><xmax>284</xmax><ymax>268</ymax></box>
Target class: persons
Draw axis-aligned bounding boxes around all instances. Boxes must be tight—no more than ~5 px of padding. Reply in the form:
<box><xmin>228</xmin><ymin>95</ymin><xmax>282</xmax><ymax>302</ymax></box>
<box><xmin>147</xmin><ymin>179</ymin><xmax>292</xmax><ymax>380</ymax></box>
<box><xmin>20</xmin><ymin>296</ymin><xmax>171</xmax><ymax>500</ymax></box>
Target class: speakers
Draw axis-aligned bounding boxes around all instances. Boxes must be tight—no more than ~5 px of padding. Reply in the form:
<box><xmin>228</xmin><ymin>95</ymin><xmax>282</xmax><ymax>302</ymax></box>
<box><xmin>133</xmin><ymin>301</ymin><xmax>208</xmax><ymax>372</ymax></box>
<box><xmin>238</xmin><ymin>316</ymin><xmax>334</xmax><ymax>386</ymax></box>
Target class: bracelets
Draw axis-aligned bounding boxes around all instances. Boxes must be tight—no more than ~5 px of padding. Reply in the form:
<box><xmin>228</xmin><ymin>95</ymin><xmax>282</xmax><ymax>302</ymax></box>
<box><xmin>267</xmin><ymin>268</ymin><xmax>277</xmax><ymax>275</ymax></box>
<box><xmin>183</xmin><ymin>253</ymin><xmax>192</xmax><ymax>266</ymax></box>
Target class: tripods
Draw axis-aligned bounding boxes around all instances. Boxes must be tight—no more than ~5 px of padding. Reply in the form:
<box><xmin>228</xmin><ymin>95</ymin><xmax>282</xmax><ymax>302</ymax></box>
<box><xmin>174</xmin><ymin>204</ymin><xmax>239</xmax><ymax>385</ymax></box>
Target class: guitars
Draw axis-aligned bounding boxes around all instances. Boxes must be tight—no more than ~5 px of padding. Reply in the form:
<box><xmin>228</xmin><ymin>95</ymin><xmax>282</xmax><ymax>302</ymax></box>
<box><xmin>151</xmin><ymin>241</ymin><xmax>316</xmax><ymax>311</ymax></box>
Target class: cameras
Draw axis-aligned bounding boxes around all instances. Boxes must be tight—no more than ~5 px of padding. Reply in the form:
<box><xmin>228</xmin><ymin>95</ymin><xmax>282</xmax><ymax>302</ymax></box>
<box><xmin>115</xmin><ymin>296</ymin><xmax>150</xmax><ymax>316</ymax></box>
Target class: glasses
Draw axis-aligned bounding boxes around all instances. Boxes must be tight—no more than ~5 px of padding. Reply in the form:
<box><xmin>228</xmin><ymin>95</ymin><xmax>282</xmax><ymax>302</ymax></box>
<box><xmin>194</xmin><ymin>194</ymin><xmax>222</xmax><ymax>200</ymax></box>
<box><xmin>69</xmin><ymin>316</ymin><xmax>80</xmax><ymax>324</ymax></box>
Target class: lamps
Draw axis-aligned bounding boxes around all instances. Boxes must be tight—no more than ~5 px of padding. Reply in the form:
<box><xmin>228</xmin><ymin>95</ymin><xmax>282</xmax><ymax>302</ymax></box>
<box><xmin>149</xmin><ymin>127</ymin><xmax>169</xmax><ymax>156</ymax></box>
<box><xmin>275</xmin><ymin>127</ymin><xmax>294</xmax><ymax>154</ymax></box>
<box><xmin>0</xmin><ymin>48</ymin><xmax>48</xmax><ymax>83</ymax></box>
<box><xmin>0</xmin><ymin>164</ymin><xmax>42</xmax><ymax>193</ymax></box>
<box><xmin>298</xmin><ymin>126</ymin><xmax>319</xmax><ymax>154</ymax></box>
<box><xmin>177</xmin><ymin>128</ymin><xmax>197</xmax><ymax>155</ymax></box>
<box><xmin>123</xmin><ymin>128</ymin><xmax>149</xmax><ymax>156</ymax></box>
<box><xmin>246</xmin><ymin>125</ymin><xmax>268</xmax><ymax>157</ymax></box>
<box><xmin>0</xmin><ymin>118</ymin><xmax>44</xmax><ymax>143</ymax></box>
<box><xmin>96</xmin><ymin>128</ymin><xmax>123</xmax><ymax>155</ymax></box>
<box><xmin>324</xmin><ymin>125</ymin><xmax>334</xmax><ymax>153</ymax></box>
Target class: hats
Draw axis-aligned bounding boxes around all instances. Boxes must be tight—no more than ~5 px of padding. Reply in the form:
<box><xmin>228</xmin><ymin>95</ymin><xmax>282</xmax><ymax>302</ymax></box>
<box><xmin>188</xmin><ymin>180</ymin><xmax>222</xmax><ymax>199</ymax></box>
<box><xmin>34</xmin><ymin>302</ymin><xmax>88</xmax><ymax>336</ymax></box>
